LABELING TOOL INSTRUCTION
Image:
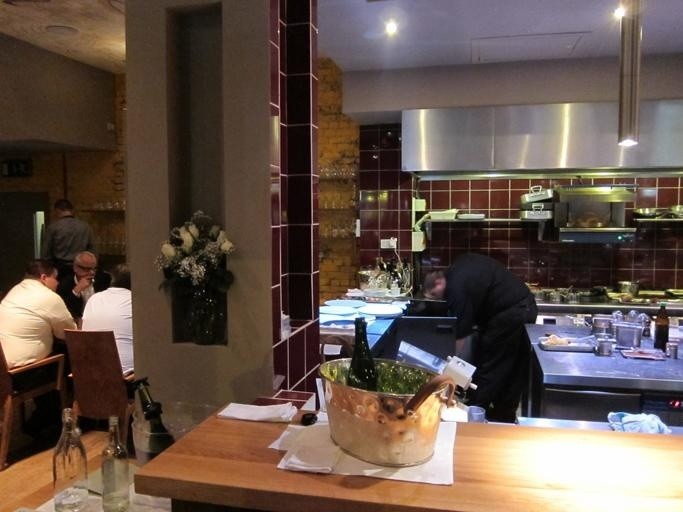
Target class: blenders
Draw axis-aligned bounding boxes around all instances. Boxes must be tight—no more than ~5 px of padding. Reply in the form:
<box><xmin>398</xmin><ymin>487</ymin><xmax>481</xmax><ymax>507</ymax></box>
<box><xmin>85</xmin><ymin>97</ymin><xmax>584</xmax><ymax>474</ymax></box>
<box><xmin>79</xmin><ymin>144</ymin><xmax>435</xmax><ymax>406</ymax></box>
<box><xmin>395</xmin><ymin>341</ymin><xmax>477</xmax><ymax>423</ymax></box>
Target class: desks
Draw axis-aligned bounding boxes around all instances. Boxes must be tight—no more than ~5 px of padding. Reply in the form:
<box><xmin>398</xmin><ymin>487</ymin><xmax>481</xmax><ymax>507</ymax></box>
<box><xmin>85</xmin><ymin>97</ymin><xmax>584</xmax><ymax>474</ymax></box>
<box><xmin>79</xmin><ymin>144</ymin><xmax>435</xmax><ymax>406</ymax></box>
<box><xmin>134</xmin><ymin>402</ymin><xmax>683</xmax><ymax>512</ymax></box>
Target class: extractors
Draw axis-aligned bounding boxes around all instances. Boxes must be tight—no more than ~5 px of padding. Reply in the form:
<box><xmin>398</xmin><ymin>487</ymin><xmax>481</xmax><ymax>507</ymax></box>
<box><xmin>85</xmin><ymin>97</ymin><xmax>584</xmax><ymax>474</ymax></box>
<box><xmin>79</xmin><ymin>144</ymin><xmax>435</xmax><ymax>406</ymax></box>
<box><xmin>541</xmin><ymin>232</ymin><xmax>635</xmax><ymax>246</ymax></box>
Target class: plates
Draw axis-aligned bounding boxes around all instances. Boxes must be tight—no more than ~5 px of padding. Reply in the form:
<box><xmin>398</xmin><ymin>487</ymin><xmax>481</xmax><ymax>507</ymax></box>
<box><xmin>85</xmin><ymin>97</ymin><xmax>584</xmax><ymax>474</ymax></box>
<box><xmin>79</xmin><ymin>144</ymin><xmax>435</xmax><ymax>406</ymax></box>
<box><xmin>318</xmin><ymin>299</ymin><xmax>365</xmax><ymax>322</ymax></box>
<box><xmin>358</xmin><ymin>305</ymin><xmax>403</xmax><ymax>316</ymax></box>
<box><xmin>457</xmin><ymin>213</ymin><xmax>484</xmax><ymax>220</ymax></box>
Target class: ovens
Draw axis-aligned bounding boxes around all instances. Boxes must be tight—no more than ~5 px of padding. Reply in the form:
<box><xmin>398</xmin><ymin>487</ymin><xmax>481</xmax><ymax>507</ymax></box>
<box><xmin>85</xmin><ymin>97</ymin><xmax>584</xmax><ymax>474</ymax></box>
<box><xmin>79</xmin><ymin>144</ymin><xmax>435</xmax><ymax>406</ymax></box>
<box><xmin>533</xmin><ymin>312</ymin><xmax>683</xmax><ymax>329</ymax></box>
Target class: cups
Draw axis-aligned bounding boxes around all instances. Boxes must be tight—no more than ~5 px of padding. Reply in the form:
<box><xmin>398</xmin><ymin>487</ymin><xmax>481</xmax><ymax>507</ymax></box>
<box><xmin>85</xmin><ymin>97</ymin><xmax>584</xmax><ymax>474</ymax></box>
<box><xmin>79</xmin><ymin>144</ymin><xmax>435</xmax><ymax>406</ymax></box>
<box><xmin>92</xmin><ymin>198</ymin><xmax>125</xmax><ymax>210</ymax></box>
<box><xmin>466</xmin><ymin>405</ymin><xmax>485</xmax><ymax>421</ymax></box>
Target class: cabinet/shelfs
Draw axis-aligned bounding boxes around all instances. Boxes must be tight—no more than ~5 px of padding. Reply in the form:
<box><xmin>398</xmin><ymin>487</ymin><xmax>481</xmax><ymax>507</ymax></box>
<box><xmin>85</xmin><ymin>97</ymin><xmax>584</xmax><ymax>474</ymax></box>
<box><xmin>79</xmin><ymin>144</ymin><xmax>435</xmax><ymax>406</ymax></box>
<box><xmin>319</xmin><ymin>175</ymin><xmax>355</xmax><ymax>238</ymax></box>
<box><xmin>82</xmin><ymin>208</ymin><xmax>125</xmax><ymax>260</ymax></box>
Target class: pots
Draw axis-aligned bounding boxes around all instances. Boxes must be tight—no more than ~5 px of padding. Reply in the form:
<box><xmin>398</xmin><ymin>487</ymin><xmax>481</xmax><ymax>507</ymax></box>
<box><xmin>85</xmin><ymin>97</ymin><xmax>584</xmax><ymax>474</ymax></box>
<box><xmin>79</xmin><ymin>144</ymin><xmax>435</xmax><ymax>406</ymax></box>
<box><xmin>631</xmin><ymin>207</ymin><xmax>679</xmax><ymax>219</ymax></box>
<box><xmin>671</xmin><ymin>205</ymin><xmax>683</xmax><ymax>219</ymax></box>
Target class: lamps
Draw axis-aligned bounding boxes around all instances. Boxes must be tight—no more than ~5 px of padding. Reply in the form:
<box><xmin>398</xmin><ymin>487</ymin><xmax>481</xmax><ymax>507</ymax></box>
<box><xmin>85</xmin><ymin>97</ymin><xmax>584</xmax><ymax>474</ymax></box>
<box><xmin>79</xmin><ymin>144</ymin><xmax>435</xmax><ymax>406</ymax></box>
<box><xmin>618</xmin><ymin>0</ymin><xmax>643</xmax><ymax>148</ymax></box>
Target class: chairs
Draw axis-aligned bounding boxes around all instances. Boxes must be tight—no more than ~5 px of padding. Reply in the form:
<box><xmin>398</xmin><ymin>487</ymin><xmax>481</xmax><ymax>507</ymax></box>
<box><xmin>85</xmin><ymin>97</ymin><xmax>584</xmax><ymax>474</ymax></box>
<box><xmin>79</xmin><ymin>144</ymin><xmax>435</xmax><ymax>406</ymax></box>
<box><xmin>64</xmin><ymin>330</ymin><xmax>138</xmax><ymax>455</ymax></box>
<box><xmin>0</xmin><ymin>342</ymin><xmax>65</xmax><ymax>473</ymax></box>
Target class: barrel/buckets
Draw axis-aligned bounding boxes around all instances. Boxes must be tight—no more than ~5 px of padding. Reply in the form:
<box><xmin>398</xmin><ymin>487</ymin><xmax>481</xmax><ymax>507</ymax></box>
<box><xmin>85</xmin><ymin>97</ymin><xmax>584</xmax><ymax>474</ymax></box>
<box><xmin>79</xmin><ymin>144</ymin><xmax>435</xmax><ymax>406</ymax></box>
<box><xmin>317</xmin><ymin>333</ymin><xmax>456</xmax><ymax>469</ymax></box>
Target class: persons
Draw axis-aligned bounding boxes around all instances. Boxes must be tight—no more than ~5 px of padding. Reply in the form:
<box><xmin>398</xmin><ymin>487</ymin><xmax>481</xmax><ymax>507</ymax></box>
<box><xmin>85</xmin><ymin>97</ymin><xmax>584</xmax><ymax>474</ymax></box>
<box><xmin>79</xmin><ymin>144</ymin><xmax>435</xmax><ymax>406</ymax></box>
<box><xmin>43</xmin><ymin>197</ymin><xmax>96</xmax><ymax>288</ymax></box>
<box><xmin>80</xmin><ymin>259</ymin><xmax>133</xmax><ymax>376</ymax></box>
<box><xmin>423</xmin><ymin>252</ymin><xmax>538</xmax><ymax>420</ymax></box>
<box><xmin>61</xmin><ymin>251</ymin><xmax>111</xmax><ymax>315</ymax></box>
<box><xmin>1</xmin><ymin>258</ymin><xmax>77</xmax><ymax>444</ymax></box>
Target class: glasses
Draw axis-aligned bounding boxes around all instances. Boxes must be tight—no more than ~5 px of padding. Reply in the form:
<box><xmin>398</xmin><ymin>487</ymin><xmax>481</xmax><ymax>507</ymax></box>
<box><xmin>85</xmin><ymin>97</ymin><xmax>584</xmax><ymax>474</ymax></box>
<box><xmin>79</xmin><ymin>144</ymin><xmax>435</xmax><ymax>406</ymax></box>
<box><xmin>78</xmin><ymin>265</ymin><xmax>96</xmax><ymax>271</ymax></box>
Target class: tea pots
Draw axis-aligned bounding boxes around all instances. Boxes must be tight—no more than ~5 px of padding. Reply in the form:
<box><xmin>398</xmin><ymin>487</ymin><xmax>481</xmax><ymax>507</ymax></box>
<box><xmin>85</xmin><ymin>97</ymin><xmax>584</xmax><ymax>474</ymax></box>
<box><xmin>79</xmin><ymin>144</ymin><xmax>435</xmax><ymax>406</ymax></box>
<box><xmin>534</xmin><ymin>288</ymin><xmax>578</xmax><ymax>304</ymax></box>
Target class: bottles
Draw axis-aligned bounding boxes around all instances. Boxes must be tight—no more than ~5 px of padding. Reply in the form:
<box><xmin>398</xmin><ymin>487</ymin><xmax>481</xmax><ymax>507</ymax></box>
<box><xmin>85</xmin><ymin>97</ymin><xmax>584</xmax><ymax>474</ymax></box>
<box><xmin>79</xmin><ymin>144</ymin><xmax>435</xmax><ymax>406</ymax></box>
<box><xmin>665</xmin><ymin>342</ymin><xmax>677</xmax><ymax>358</ymax></box>
<box><xmin>100</xmin><ymin>416</ymin><xmax>130</xmax><ymax>512</ymax></box>
<box><xmin>655</xmin><ymin>302</ymin><xmax>670</xmax><ymax>349</ymax></box>
<box><xmin>348</xmin><ymin>318</ymin><xmax>376</xmax><ymax>393</ymax></box>
<box><xmin>613</xmin><ymin>309</ymin><xmax>650</xmax><ymax>336</ymax></box>
<box><xmin>50</xmin><ymin>407</ymin><xmax>87</xmax><ymax>511</ymax></box>
<box><xmin>134</xmin><ymin>380</ymin><xmax>175</xmax><ymax>457</ymax></box>
<box><xmin>367</xmin><ymin>257</ymin><xmax>411</xmax><ymax>294</ymax></box>
<box><xmin>595</xmin><ymin>338</ymin><xmax>614</xmax><ymax>355</ymax></box>
<box><xmin>538</xmin><ymin>260</ymin><xmax>549</xmax><ymax>288</ymax></box>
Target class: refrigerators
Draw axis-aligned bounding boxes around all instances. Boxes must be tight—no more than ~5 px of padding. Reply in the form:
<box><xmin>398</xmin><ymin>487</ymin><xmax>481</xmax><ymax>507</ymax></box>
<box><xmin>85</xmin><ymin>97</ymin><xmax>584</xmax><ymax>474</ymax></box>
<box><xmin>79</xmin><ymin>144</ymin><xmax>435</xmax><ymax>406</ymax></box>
<box><xmin>538</xmin><ymin>382</ymin><xmax>683</xmax><ymax>428</ymax></box>
